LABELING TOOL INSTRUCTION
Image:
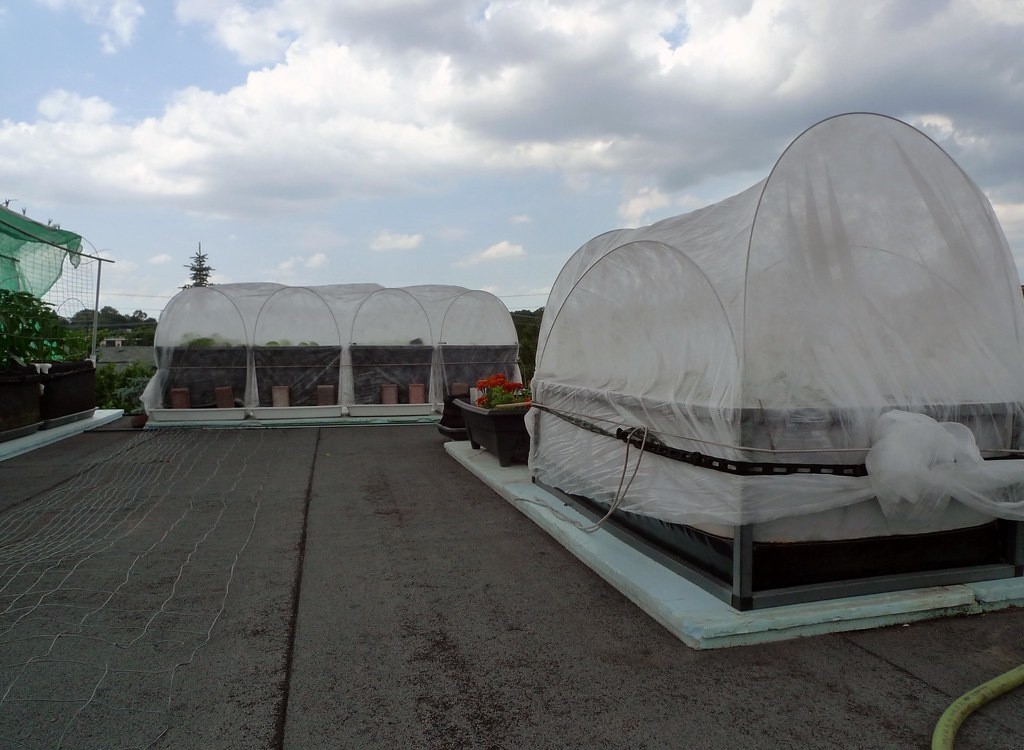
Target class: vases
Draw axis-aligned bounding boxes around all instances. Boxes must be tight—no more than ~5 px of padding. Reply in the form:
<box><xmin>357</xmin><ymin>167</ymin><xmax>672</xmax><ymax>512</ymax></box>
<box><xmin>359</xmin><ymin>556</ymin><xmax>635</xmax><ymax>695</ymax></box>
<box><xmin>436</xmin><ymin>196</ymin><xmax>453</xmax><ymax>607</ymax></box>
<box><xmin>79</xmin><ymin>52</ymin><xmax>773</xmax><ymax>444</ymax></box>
<box><xmin>452</xmin><ymin>397</ymin><xmax>530</xmax><ymax>467</ymax></box>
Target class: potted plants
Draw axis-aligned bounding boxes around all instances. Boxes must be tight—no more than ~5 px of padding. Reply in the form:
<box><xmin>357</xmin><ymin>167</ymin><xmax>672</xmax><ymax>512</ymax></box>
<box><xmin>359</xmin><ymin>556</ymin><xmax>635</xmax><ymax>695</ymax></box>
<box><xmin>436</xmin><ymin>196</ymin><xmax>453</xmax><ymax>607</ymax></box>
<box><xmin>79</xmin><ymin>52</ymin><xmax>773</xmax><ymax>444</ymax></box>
<box><xmin>130</xmin><ymin>405</ymin><xmax>149</xmax><ymax>428</ymax></box>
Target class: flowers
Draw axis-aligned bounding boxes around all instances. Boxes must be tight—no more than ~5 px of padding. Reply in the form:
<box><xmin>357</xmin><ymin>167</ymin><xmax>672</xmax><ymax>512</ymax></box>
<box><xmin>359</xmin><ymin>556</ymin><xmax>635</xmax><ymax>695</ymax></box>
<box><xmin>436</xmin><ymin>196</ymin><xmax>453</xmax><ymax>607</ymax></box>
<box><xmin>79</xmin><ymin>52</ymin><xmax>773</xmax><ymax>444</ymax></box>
<box><xmin>474</xmin><ymin>372</ymin><xmax>532</xmax><ymax>411</ymax></box>
